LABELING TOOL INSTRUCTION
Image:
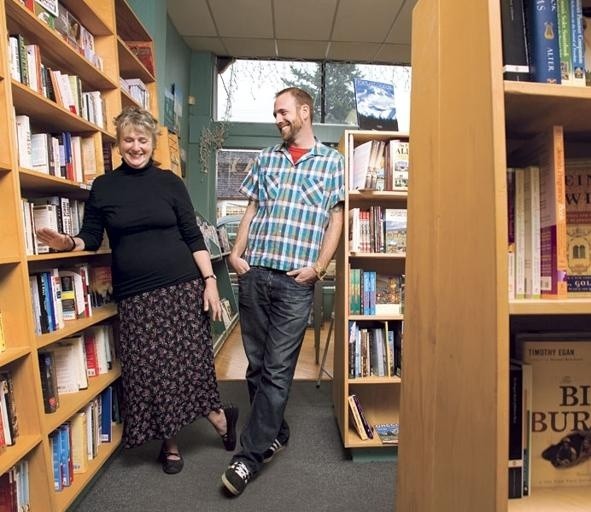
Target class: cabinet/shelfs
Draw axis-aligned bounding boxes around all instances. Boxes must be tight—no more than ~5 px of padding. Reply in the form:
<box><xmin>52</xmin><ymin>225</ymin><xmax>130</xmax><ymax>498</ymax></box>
<box><xmin>199</xmin><ymin>248</ymin><xmax>244</xmax><ymax>361</ymax></box>
<box><xmin>389</xmin><ymin>0</ymin><xmax>590</xmax><ymax>512</ymax></box>
<box><xmin>0</xmin><ymin>0</ymin><xmax>183</xmax><ymax>512</ymax></box>
<box><xmin>333</xmin><ymin>126</ymin><xmax>409</xmax><ymax>463</ymax></box>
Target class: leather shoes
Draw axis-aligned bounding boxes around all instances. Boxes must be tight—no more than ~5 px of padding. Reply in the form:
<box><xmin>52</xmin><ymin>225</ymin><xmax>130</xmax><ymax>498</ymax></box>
<box><xmin>221</xmin><ymin>400</ymin><xmax>239</xmax><ymax>451</ymax></box>
<box><xmin>161</xmin><ymin>440</ymin><xmax>184</xmax><ymax>474</ymax></box>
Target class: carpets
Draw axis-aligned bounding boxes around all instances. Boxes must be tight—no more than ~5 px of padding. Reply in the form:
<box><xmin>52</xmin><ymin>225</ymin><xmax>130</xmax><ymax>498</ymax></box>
<box><xmin>64</xmin><ymin>376</ymin><xmax>399</xmax><ymax>512</ymax></box>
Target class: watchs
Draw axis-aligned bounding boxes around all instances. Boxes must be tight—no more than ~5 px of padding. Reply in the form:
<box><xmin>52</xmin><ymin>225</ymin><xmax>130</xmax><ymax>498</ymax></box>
<box><xmin>204</xmin><ymin>273</ymin><xmax>217</xmax><ymax>281</ymax></box>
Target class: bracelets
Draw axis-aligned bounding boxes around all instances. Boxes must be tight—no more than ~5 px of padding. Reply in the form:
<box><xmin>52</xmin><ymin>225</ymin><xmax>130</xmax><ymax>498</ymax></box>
<box><xmin>62</xmin><ymin>228</ymin><xmax>75</xmax><ymax>251</ymax></box>
<box><xmin>313</xmin><ymin>264</ymin><xmax>327</xmax><ymax>281</ymax></box>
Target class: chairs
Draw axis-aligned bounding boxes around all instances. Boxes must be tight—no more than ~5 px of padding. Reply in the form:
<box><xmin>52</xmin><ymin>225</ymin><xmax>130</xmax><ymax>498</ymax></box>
<box><xmin>311</xmin><ymin>277</ymin><xmax>333</xmax><ymax>366</ymax></box>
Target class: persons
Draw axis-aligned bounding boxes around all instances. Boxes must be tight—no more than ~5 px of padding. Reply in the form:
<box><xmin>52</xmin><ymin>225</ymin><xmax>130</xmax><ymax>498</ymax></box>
<box><xmin>220</xmin><ymin>86</ymin><xmax>346</xmax><ymax>495</ymax></box>
<box><xmin>35</xmin><ymin>104</ymin><xmax>243</xmax><ymax>476</ymax></box>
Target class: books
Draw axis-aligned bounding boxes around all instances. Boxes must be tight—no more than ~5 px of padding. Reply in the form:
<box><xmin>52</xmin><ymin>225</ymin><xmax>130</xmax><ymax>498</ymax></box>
<box><xmin>198</xmin><ymin>216</ymin><xmax>238</xmax><ymax>328</ymax></box>
<box><xmin>120</xmin><ymin>69</ymin><xmax>149</xmax><ymax>107</ymax></box>
<box><xmin>28</xmin><ymin>262</ymin><xmax>112</xmax><ymax>337</ymax></box>
<box><xmin>13</xmin><ymin>106</ymin><xmax>105</xmax><ymax>183</ymax></box>
<box><xmin>23</xmin><ymin>2</ymin><xmax>103</xmax><ymax>71</ymax></box>
<box><xmin>41</xmin><ymin>64</ymin><xmax>107</xmax><ymax>131</ymax></box>
<box><xmin>48</xmin><ymin>387</ymin><xmax>112</xmax><ymax>491</ymax></box>
<box><xmin>355</xmin><ymin>79</ymin><xmax>400</xmax><ymax>132</ymax></box>
<box><xmin>2</xmin><ymin>460</ymin><xmax>30</xmax><ymax>510</ymax></box>
<box><xmin>2</xmin><ymin>370</ymin><xmax>19</xmax><ymax>447</ymax></box>
<box><xmin>37</xmin><ymin>323</ymin><xmax>112</xmax><ymax>414</ymax></box>
<box><xmin>22</xmin><ymin>196</ymin><xmax>84</xmax><ymax>256</ymax></box>
<box><xmin>7</xmin><ymin>28</ymin><xmax>44</xmax><ymax>97</ymax></box>
<box><xmin>345</xmin><ymin>136</ymin><xmax>410</xmax><ymax>446</ymax></box>
<box><xmin>499</xmin><ymin>1</ymin><xmax>591</xmax><ymax>500</ymax></box>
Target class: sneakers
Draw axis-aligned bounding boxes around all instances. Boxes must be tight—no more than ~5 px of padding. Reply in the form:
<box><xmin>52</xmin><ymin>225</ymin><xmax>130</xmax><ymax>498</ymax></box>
<box><xmin>263</xmin><ymin>435</ymin><xmax>288</xmax><ymax>465</ymax></box>
<box><xmin>222</xmin><ymin>459</ymin><xmax>252</xmax><ymax>496</ymax></box>
